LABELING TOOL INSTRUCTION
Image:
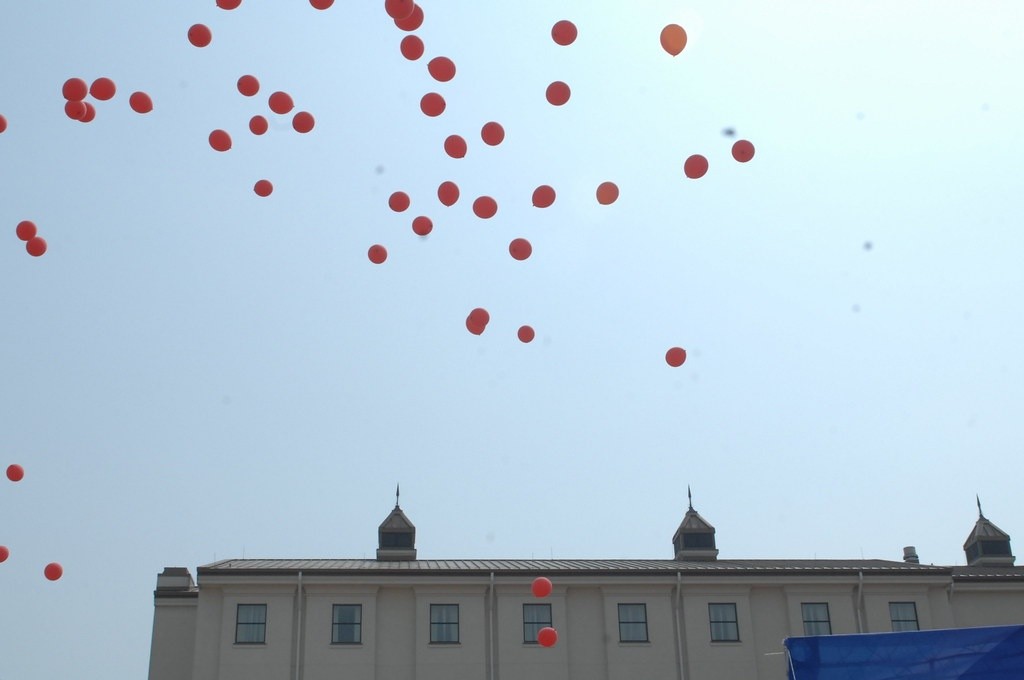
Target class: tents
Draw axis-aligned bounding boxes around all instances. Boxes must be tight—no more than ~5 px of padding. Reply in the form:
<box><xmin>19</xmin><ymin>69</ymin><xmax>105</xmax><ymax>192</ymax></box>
<box><xmin>781</xmin><ymin>623</ymin><xmax>1024</xmax><ymax>680</ymax></box>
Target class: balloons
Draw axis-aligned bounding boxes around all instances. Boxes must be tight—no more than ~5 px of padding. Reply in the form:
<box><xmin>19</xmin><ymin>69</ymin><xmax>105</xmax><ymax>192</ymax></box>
<box><xmin>0</xmin><ymin>113</ymin><xmax>7</xmax><ymax>133</ymax></box>
<box><xmin>43</xmin><ymin>562</ymin><xmax>62</xmax><ymax>581</ymax></box>
<box><xmin>684</xmin><ymin>154</ymin><xmax>709</xmax><ymax>179</ymax></box>
<box><xmin>665</xmin><ymin>347</ymin><xmax>687</xmax><ymax>368</ymax></box>
<box><xmin>531</xmin><ymin>577</ymin><xmax>552</xmax><ymax>597</ymax></box>
<box><xmin>16</xmin><ymin>0</ymin><xmax>578</xmax><ymax>264</ymax></box>
<box><xmin>659</xmin><ymin>23</ymin><xmax>688</xmax><ymax>57</ymax></box>
<box><xmin>0</xmin><ymin>545</ymin><xmax>9</xmax><ymax>563</ymax></box>
<box><xmin>6</xmin><ymin>464</ymin><xmax>24</xmax><ymax>482</ymax></box>
<box><xmin>731</xmin><ymin>140</ymin><xmax>755</xmax><ymax>163</ymax></box>
<box><xmin>596</xmin><ymin>181</ymin><xmax>619</xmax><ymax>205</ymax></box>
<box><xmin>537</xmin><ymin>627</ymin><xmax>558</xmax><ymax>647</ymax></box>
<box><xmin>466</xmin><ymin>307</ymin><xmax>490</xmax><ymax>337</ymax></box>
<box><xmin>517</xmin><ymin>325</ymin><xmax>535</xmax><ymax>343</ymax></box>
